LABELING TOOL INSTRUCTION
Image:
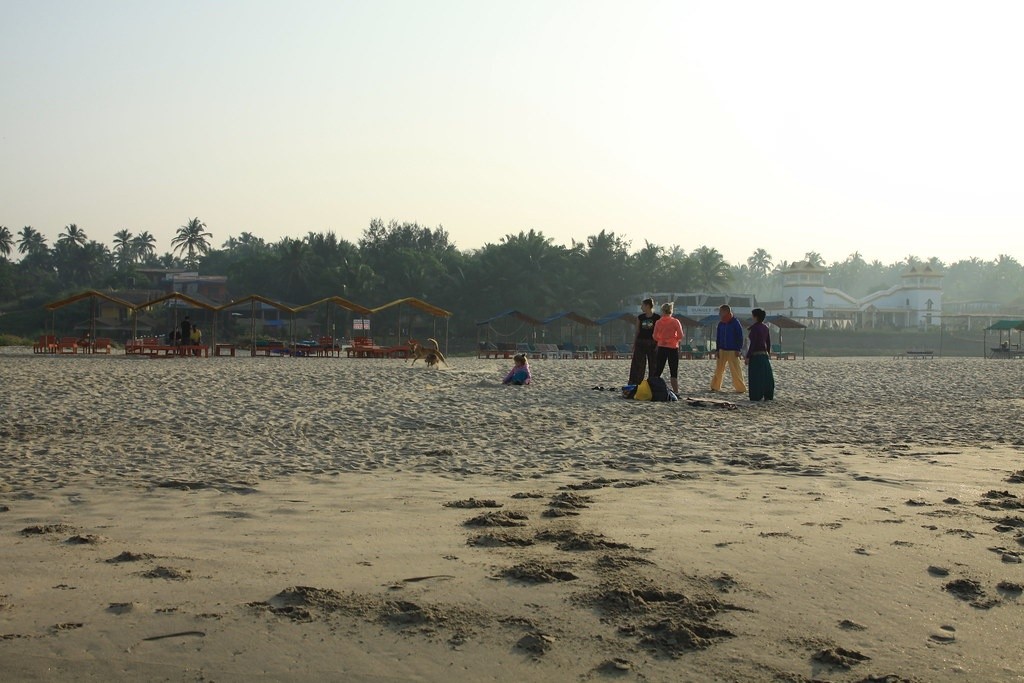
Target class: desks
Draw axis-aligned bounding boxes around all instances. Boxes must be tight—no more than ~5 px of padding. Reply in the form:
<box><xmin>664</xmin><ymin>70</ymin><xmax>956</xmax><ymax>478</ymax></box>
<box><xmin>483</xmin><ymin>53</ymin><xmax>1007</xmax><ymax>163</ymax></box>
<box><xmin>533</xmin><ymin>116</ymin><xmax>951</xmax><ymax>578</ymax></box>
<box><xmin>215</xmin><ymin>344</ymin><xmax>235</xmax><ymax>357</ymax></box>
<box><xmin>908</xmin><ymin>351</ymin><xmax>933</xmax><ymax>359</ymax></box>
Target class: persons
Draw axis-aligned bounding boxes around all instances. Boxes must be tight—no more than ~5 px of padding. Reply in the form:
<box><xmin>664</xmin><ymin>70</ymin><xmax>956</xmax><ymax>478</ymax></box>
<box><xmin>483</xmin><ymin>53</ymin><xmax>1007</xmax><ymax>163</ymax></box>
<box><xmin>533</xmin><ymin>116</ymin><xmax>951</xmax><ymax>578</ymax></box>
<box><xmin>501</xmin><ymin>352</ymin><xmax>531</xmax><ymax>388</ymax></box>
<box><xmin>625</xmin><ymin>299</ymin><xmax>657</xmax><ymax>390</ymax></box>
<box><xmin>652</xmin><ymin>300</ymin><xmax>685</xmax><ymax>398</ymax></box>
<box><xmin>190</xmin><ymin>323</ymin><xmax>202</xmax><ymax>355</ymax></box>
<box><xmin>710</xmin><ymin>304</ymin><xmax>748</xmax><ymax>393</ymax></box>
<box><xmin>169</xmin><ymin>325</ymin><xmax>182</xmax><ymax>346</ymax></box>
<box><xmin>180</xmin><ymin>316</ymin><xmax>191</xmax><ymax>357</ymax></box>
<box><xmin>743</xmin><ymin>309</ymin><xmax>776</xmax><ymax>401</ymax></box>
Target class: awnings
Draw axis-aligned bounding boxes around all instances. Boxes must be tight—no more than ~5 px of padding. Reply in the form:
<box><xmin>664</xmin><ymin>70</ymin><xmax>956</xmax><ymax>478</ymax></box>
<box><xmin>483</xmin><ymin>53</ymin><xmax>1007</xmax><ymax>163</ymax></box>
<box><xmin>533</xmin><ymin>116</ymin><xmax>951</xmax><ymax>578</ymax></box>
<box><xmin>473</xmin><ymin>300</ymin><xmax>809</xmax><ymax>358</ymax></box>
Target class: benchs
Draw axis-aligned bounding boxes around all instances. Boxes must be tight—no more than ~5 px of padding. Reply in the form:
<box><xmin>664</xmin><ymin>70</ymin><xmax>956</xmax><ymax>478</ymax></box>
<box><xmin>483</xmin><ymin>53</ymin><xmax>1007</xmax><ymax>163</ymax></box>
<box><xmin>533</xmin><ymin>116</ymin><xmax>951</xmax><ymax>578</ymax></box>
<box><xmin>179</xmin><ymin>345</ymin><xmax>209</xmax><ymax>357</ymax></box>
<box><xmin>892</xmin><ymin>353</ymin><xmax>934</xmax><ymax>360</ymax></box>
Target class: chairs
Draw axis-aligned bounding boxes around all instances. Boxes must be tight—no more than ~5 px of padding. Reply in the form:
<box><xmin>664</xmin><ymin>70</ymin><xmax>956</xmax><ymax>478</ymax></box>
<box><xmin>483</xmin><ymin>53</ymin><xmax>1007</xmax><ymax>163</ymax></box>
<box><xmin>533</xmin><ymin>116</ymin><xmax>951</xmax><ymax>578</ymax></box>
<box><xmin>95</xmin><ymin>337</ymin><xmax>111</xmax><ymax>354</ymax></box>
<box><xmin>141</xmin><ymin>337</ymin><xmax>178</xmax><ymax>355</ymax></box>
<box><xmin>33</xmin><ymin>336</ymin><xmax>57</xmax><ymax>354</ymax></box>
<box><xmin>125</xmin><ymin>339</ymin><xmax>142</xmax><ymax>355</ymax></box>
<box><xmin>256</xmin><ymin>335</ymin><xmax>412</xmax><ymax>359</ymax></box>
<box><xmin>57</xmin><ymin>336</ymin><xmax>78</xmax><ymax>354</ymax></box>
<box><xmin>478</xmin><ymin>341</ymin><xmax>796</xmax><ymax>360</ymax></box>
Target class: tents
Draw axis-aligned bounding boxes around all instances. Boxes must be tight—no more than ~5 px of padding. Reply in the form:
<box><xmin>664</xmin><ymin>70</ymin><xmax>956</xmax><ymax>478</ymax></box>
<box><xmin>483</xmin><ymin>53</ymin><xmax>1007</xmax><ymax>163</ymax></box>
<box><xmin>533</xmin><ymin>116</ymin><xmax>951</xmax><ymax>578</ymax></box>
<box><xmin>983</xmin><ymin>320</ymin><xmax>1024</xmax><ymax>359</ymax></box>
<box><xmin>43</xmin><ymin>290</ymin><xmax>453</xmax><ymax>358</ymax></box>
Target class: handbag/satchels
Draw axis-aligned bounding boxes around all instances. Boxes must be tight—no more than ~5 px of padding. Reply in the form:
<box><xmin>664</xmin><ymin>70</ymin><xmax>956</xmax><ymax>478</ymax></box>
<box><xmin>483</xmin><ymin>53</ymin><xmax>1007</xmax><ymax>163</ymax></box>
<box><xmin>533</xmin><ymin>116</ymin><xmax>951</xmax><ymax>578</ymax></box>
<box><xmin>633</xmin><ymin>378</ymin><xmax>682</xmax><ymax>401</ymax></box>
